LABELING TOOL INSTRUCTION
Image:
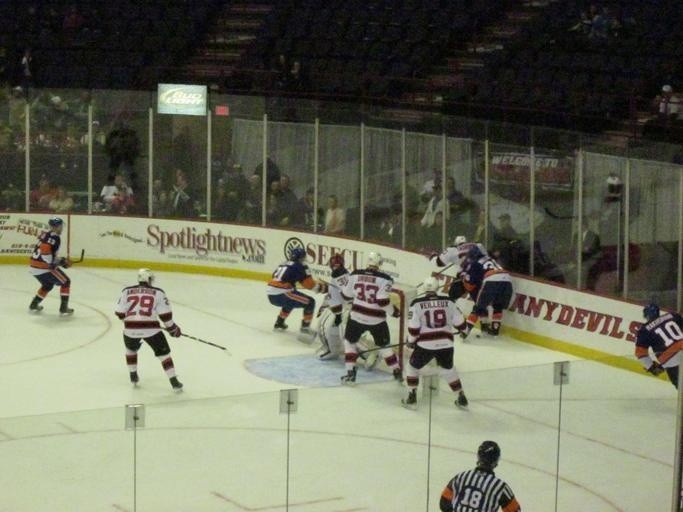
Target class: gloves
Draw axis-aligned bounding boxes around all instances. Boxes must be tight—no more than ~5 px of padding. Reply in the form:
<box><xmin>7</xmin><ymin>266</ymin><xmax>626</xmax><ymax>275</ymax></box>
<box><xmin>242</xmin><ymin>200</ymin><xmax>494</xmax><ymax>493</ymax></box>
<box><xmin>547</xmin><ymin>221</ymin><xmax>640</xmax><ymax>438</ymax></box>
<box><xmin>648</xmin><ymin>361</ymin><xmax>664</xmax><ymax>377</ymax></box>
<box><xmin>335</xmin><ymin>312</ymin><xmax>342</xmax><ymax>326</ymax></box>
<box><xmin>59</xmin><ymin>257</ymin><xmax>73</xmax><ymax>269</ymax></box>
<box><xmin>318</xmin><ymin>283</ymin><xmax>328</xmax><ymax>294</ymax></box>
<box><xmin>166</xmin><ymin>323</ymin><xmax>181</xmax><ymax>337</ymax></box>
<box><xmin>392</xmin><ymin>304</ymin><xmax>401</xmax><ymax>318</ymax></box>
<box><xmin>406</xmin><ymin>335</ymin><xmax>419</xmax><ymax>349</ymax></box>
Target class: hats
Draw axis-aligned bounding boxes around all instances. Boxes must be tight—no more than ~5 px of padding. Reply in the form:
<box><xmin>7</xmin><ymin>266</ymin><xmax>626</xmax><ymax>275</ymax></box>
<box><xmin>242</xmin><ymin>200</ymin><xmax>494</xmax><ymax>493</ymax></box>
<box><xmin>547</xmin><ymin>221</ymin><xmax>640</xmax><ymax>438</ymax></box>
<box><xmin>92</xmin><ymin>121</ymin><xmax>100</xmax><ymax>126</ymax></box>
<box><xmin>432</xmin><ymin>179</ymin><xmax>442</xmax><ymax>188</ymax></box>
<box><xmin>497</xmin><ymin>213</ymin><xmax>511</xmax><ymax>221</ymax></box>
<box><xmin>306</xmin><ymin>186</ymin><xmax>321</xmax><ymax>195</ymax></box>
<box><xmin>47</xmin><ymin>96</ymin><xmax>61</xmax><ymax>105</ymax></box>
<box><xmin>12</xmin><ymin>85</ymin><xmax>24</xmax><ymax>95</ymax></box>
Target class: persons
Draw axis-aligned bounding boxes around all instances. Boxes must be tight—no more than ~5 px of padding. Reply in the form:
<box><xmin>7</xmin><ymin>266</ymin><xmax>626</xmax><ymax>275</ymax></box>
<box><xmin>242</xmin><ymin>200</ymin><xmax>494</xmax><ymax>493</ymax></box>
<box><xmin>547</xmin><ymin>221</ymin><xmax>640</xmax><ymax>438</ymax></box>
<box><xmin>214</xmin><ymin>155</ymin><xmax>320</xmax><ymax>232</ymax></box>
<box><xmin>565</xmin><ymin>3</ymin><xmax>621</xmax><ymax>54</ymax></box>
<box><xmin>634</xmin><ymin>304</ymin><xmax>682</xmax><ymax>388</ymax></box>
<box><xmin>570</xmin><ymin>216</ymin><xmax>600</xmax><ymax>263</ymax></box>
<box><xmin>88</xmin><ymin>170</ymin><xmax>135</xmax><ymax>216</ymax></box>
<box><xmin>1</xmin><ymin>171</ymin><xmax>73</xmax><ymax>214</ymax></box>
<box><xmin>429</xmin><ymin>235</ymin><xmax>490</xmax><ymax>335</ymax></box>
<box><xmin>146</xmin><ymin>168</ymin><xmax>196</xmax><ymax>220</ymax></box>
<box><xmin>0</xmin><ymin>46</ymin><xmax>107</xmax><ymax>171</ymax></box>
<box><xmin>439</xmin><ymin>441</ymin><xmax>520</xmax><ymax>511</ymax></box>
<box><xmin>340</xmin><ymin>251</ymin><xmax>402</xmax><ymax>385</ymax></box>
<box><xmin>458</xmin><ymin>247</ymin><xmax>514</xmax><ymax>340</ymax></box>
<box><xmin>315</xmin><ymin>253</ymin><xmax>351</xmax><ymax>360</ymax></box>
<box><xmin>471</xmin><ymin>211</ymin><xmax>565</xmax><ymax>285</ymax></box>
<box><xmin>381</xmin><ymin>170</ymin><xmax>479</xmax><ymax>251</ymax></box>
<box><xmin>28</xmin><ymin>218</ymin><xmax>76</xmax><ymax>314</ymax></box>
<box><xmin>114</xmin><ymin>267</ymin><xmax>186</xmax><ymax>389</ymax></box>
<box><xmin>400</xmin><ymin>277</ymin><xmax>469</xmax><ymax>410</ymax></box>
<box><xmin>658</xmin><ymin>81</ymin><xmax>672</xmax><ymax>117</ymax></box>
<box><xmin>322</xmin><ymin>195</ymin><xmax>346</xmax><ymax>235</ymax></box>
<box><xmin>266</xmin><ymin>247</ymin><xmax>328</xmax><ymax>335</ymax></box>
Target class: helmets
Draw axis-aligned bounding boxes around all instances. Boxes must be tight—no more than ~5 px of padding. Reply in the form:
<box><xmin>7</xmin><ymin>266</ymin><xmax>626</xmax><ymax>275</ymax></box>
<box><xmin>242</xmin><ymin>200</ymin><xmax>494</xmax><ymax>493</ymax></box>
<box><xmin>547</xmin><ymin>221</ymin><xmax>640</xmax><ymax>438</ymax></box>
<box><xmin>137</xmin><ymin>268</ymin><xmax>154</xmax><ymax>286</ymax></box>
<box><xmin>424</xmin><ymin>277</ymin><xmax>439</xmax><ymax>292</ymax></box>
<box><xmin>330</xmin><ymin>256</ymin><xmax>344</xmax><ymax>271</ymax></box>
<box><xmin>367</xmin><ymin>252</ymin><xmax>383</xmax><ymax>269</ymax></box>
<box><xmin>477</xmin><ymin>441</ymin><xmax>500</xmax><ymax>469</ymax></box>
<box><xmin>48</xmin><ymin>217</ymin><xmax>66</xmax><ymax>235</ymax></box>
<box><xmin>469</xmin><ymin>243</ymin><xmax>488</xmax><ymax>260</ymax></box>
<box><xmin>289</xmin><ymin>248</ymin><xmax>306</xmax><ymax>261</ymax></box>
<box><xmin>463</xmin><ymin>274</ymin><xmax>478</xmax><ymax>292</ymax></box>
<box><xmin>643</xmin><ymin>303</ymin><xmax>660</xmax><ymax>322</ymax></box>
<box><xmin>454</xmin><ymin>235</ymin><xmax>466</xmax><ymax>246</ymax></box>
<box><xmin>606</xmin><ymin>176</ymin><xmax>622</xmax><ymax>198</ymax></box>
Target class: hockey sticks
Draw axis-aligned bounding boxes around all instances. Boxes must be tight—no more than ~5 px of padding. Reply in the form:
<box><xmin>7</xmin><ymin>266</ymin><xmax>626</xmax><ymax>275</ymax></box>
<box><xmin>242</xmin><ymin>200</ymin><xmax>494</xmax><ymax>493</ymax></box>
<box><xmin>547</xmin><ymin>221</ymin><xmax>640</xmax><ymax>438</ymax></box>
<box><xmin>70</xmin><ymin>249</ymin><xmax>84</xmax><ymax>263</ymax></box>
<box><xmin>157</xmin><ymin>326</ymin><xmax>233</xmax><ymax>356</ymax></box>
<box><xmin>358</xmin><ymin>331</ymin><xmax>460</xmax><ymax>360</ymax></box>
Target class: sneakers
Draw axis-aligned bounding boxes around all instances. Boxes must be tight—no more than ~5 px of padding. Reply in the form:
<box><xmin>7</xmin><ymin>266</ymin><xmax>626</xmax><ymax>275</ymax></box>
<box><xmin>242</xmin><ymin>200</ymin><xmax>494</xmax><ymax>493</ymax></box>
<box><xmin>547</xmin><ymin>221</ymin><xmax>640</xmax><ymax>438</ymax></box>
<box><xmin>455</xmin><ymin>395</ymin><xmax>468</xmax><ymax>406</ymax></box>
<box><xmin>60</xmin><ymin>303</ymin><xmax>74</xmax><ymax>313</ymax></box>
<box><xmin>169</xmin><ymin>376</ymin><xmax>183</xmax><ymax>388</ymax></box>
<box><xmin>392</xmin><ymin>368</ymin><xmax>403</xmax><ymax>380</ymax></box>
<box><xmin>401</xmin><ymin>392</ymin><xmax>417</xmax><ymax>405</ymax></box>
<box><xmin>319</xmin><ymin>351</ymin><xmax>339</xmax><ymax>360</ymax></box>
<box><xmin>29</xmin><ymin>301</ymin><xmax>43</xmax><ymax>311</ymax></box>
<box><xmin>300</xmin><ymin>319</ymin><xmax>311</xmax><ymax>333</ymax></box>
<box><xmin>459</xmin><ymin>321</ymin><xmax>474</xmax><ymax>337</ymax></box>
<box><xmin>476</xmin><ymin>320</ymin><xmax>490</xmax><ymax>338</ymax></box>
<box><xmin>488</xmin><ymin>321</ymin><xmax>501</xmax><ymax>336</ymax></box>
<box><xmin>274</xmin><ymin>315</ymin><xmax>288</xmax><ymax>329</ymax></box>
<box><xmin>340</xmin><ymin>366</ymin><xmax>357</xmax><ymax>382</ymax></box>
<box><xmin>366</xmin><ymin>349</ymin><xmax>380</xmax><ymax>369</ymax></box>
<box><xmin>130</xmin><ymin>371</ymin><xmax>139</xmax><ymax>382</ymax></box>
